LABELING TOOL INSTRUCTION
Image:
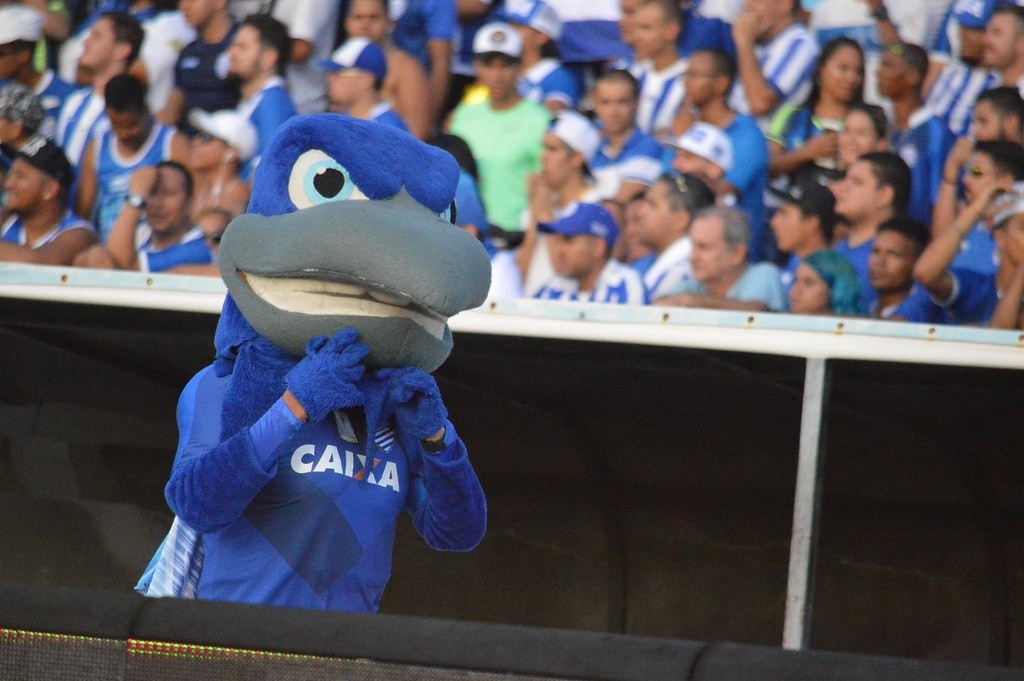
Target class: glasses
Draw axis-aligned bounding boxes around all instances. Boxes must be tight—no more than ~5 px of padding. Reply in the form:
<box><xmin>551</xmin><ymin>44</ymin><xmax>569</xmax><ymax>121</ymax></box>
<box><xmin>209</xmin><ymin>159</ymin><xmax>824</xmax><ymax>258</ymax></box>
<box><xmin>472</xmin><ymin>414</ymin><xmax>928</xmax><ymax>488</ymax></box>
<box><xmin>192</xmin><ymin>132</ymin><xmax>212</xmax><ymax>142</ymax></box>
<box><xmin>886</xmin><ymin>40</ymin><xmax>908</xmax><ymax>55</ymax></box>
<box><xmin>336</xmin><ymin>70</ymin><xmax>371</xmax><ymax>78</ymax></box>
<box><xmin>682</xmin><ymin>69</ymin><xmax>721</xmax><ymax>79</ymax></box>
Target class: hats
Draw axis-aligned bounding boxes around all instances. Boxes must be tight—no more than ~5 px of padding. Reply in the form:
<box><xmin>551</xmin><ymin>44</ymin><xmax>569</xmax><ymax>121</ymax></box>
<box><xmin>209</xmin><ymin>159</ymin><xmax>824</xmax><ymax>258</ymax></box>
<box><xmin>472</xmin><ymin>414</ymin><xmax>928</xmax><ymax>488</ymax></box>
<box><xmin>618</xmin><ymin>154</ymin><xmax>664</xmax><ymax>188</ymax></box>
<box><xmin>494</xmin><ymin>0</ymin><xmax>564</xmax><ymax>42</ymax></box>
<box><xmin>472</xmin><ymin>21</ymin><xmax>524</xmax><ymax>57</ymax></box>
<box><xmin>187</xmin><ymin>106</ymin><xmax>259</xmax><ymax>163</ymax></box>
<box><xmin>940</xmin><ymin>1</ymin><xmax>995</xmax><ymax>27</ymax></box>
<box><xmin>763</xmin><ymin>180</ymin><xmax>835</xmax><ymax>217</ymax></box>
<box><xmin>988</xmin><ymin>181</ymin><xmax>1024</xmax><ymax>239</ymax></box>
<box><xmin>0</xmin><ymin>81</ymin><xmax>46</xmax><ymax>130</ymax></box>
<box><xmin>547</xmin><ymin>109</ymin><xmax>602</xmax><ymax>183</ymax></box>
<box><xmin>1</xmin><ymin>7</ymin><xmax>46</xmax><ymax>47</ymax></box>
<box><xmin>536</xmin><ymin>202</ymin><xmax>619</xmax><ymax>245</ymax></box>
<box><xmin>661</xmin><ymin>120</ymin><xmax>733</xmax><ymax>172</ymax></box>
<box><xmin>0</xmin><ymin>135</ymin><xmax>74</xmax><ymax>189</ymax></box>
<box><xmin>318</xmin><ymin>36</ymin><xmax>387</xmax><ymax>76</ymax></box>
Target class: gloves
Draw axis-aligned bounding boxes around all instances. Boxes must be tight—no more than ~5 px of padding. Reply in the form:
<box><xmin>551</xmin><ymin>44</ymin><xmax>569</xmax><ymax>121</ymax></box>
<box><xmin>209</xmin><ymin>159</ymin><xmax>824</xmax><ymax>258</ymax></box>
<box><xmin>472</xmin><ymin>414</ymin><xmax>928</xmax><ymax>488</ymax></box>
<box><xmin>285</xmin><ymin>326</ymin><xmax>371</xmax><ymax>423</ymax></box>
<box><xmin>374</xmin><ymin>368</ymin><xmax>448</xmax><ymax>440</ymax></box>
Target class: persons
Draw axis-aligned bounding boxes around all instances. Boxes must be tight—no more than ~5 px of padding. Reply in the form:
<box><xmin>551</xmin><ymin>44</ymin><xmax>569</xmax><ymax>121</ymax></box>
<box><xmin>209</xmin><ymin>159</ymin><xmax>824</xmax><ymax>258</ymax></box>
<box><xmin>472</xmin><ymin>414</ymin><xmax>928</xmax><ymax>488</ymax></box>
<box><xmin>136</xmin><ymin>113</ymin><xmax>491</xmax><ymax>615</ymax></box>
<box><xmin>0</xmin><ymin>0</ymin><xmax>1024</xmax><ymax>336</ymax></box>
